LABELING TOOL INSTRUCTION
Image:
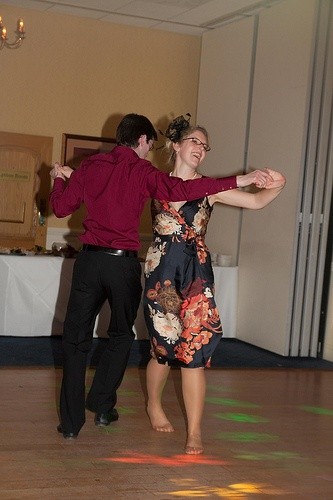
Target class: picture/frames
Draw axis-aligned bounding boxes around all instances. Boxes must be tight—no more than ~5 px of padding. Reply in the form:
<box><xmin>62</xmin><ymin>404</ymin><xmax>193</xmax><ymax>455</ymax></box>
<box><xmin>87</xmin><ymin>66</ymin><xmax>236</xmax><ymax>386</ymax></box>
<box><xmin>61</xmin><ymin>134</ymin><xmax>118</xmax><ymax>171</ymax></box>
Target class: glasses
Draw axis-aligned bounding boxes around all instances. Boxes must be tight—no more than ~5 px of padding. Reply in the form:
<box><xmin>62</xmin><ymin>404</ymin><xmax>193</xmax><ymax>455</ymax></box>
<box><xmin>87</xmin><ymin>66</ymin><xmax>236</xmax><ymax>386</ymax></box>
<box><xmin>178</xmin><ymin>137</ymin><xmax>211</xmax><ymax>151</ymax></box>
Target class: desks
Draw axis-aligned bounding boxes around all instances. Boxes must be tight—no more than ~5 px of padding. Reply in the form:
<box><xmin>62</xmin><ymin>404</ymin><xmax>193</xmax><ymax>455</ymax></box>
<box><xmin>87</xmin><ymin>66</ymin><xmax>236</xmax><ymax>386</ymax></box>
<box><xmin>0</xmin><ymin>253</ymin><xmax>238</xmax><ymax>338</ymax></box>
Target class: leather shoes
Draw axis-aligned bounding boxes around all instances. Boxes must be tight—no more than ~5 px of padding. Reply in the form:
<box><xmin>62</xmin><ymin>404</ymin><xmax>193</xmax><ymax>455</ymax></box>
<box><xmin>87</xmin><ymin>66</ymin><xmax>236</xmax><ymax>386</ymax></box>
<box><xmin>95</xmin><ymin>411</ymin><xmax>119</xmax><ymax>426</ymax></box>
<box><xmin>63</xmin><ymin>430</ymin><xmax>79</xmax><ymax>439</ymax></box>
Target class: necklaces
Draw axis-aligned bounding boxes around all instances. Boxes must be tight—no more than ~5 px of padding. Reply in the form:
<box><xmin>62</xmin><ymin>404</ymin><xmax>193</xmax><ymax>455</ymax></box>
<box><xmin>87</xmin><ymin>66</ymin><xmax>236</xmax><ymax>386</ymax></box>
<box><xmin>170</xmin><ymin>170</ymin><xmax>198</xmax><ymax>180</ymax></box>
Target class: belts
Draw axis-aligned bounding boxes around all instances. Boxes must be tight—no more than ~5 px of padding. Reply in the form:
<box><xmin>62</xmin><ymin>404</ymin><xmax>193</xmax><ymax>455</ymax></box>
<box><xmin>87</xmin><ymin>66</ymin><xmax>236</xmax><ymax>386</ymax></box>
<box><xmin>84</xmin><ymin>244</ymin><xmax>135</xmax><ymax>257</ymax></box>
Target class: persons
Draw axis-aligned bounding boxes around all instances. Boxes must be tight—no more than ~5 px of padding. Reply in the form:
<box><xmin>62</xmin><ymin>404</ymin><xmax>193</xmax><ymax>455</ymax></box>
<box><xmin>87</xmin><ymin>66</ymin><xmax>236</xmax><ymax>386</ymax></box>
<box><xmin>49</xmin><ymin>113</ymin><xmax>286</xmax><ymax>456</ymax></box>
<box><xmin>48</xmin><ymin>113</ymin><xmax>272</xmax><ymax>440</ymax></box>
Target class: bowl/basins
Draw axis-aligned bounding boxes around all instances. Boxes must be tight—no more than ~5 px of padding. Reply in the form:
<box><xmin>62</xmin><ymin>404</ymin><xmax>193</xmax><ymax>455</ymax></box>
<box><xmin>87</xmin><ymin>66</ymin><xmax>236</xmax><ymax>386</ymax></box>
<box><xmin>217</xmin><ymin>254</ymin><xmax>232</xmax><ymax>266</ymax></box>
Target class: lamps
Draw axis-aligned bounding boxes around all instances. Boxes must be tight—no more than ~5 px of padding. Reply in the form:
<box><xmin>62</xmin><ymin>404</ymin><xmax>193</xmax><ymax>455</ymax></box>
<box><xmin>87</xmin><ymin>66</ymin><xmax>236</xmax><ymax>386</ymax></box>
<box><xmin>0</xmin><ymin>16</ymin><xmax>26</xmax><ymax>50</ymax></box>
